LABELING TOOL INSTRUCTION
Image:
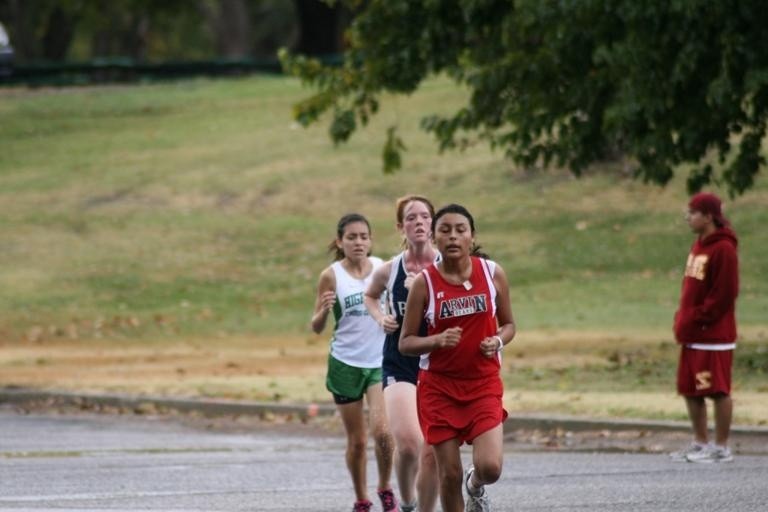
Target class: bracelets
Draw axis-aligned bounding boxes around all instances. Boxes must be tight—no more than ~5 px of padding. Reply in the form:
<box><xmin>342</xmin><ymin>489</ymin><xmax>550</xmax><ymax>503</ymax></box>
<box><xmin>493</xmin><ymin>335</ymin><xmax>503</xmax><ymax>351</ymax></box>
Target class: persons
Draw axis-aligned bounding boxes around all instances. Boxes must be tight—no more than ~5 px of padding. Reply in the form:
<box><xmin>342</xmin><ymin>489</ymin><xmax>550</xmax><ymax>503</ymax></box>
<box><xmin>312</xmin><ymin>215</ymin><xmax>397</xmax><ymax>512</ymax></box>
<box><xmin>363</xmin><ymin>195</ymin><xmax>443</xmax><ymax>512</ymax></box>
<box><xmin>670</xmin><ymin>193</ymin><xmax>739</xmax><ymax>462</ymax></box>
<box><xmin>397</xmin><ymin>204</ymin><xmax>515</xmax><ymax>512</ymax></box>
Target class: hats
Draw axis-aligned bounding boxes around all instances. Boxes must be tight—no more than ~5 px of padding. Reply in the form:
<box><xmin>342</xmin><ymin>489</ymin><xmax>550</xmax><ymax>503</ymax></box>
<box><xmin>690</xmin><ymin>193</ymin><xmax>732</xmax><ymax>227</ymax></box>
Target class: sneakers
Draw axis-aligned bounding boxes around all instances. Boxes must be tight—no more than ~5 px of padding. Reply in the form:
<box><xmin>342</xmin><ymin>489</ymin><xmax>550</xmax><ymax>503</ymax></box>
<box><xmin>377</xmin><ymin>485</ymin><xmax>399</xmax><ymax>512</ymax></box>
<box><xmin>462</xmin><ymin>468</ymin><xmax>489</xmax><ymax>512</ymax></box>
<box><xmin>686</xmin><ymin>441</ymin><xmax>733</xmax><ymax>465</ymax></box>
<box><xmin>399</xmin><ymin>499</ymin><xmax>418</xmax><ymax>512</ymax></box>
<box><xmin>669</xmin><ymin>440</ymin><xmax>703</xmax><ymax>463</ymax></box>
<box><xmin>352</xmin><ymin>498</ymin><xmax>372</xmax><ymax>512</ymax></box>
